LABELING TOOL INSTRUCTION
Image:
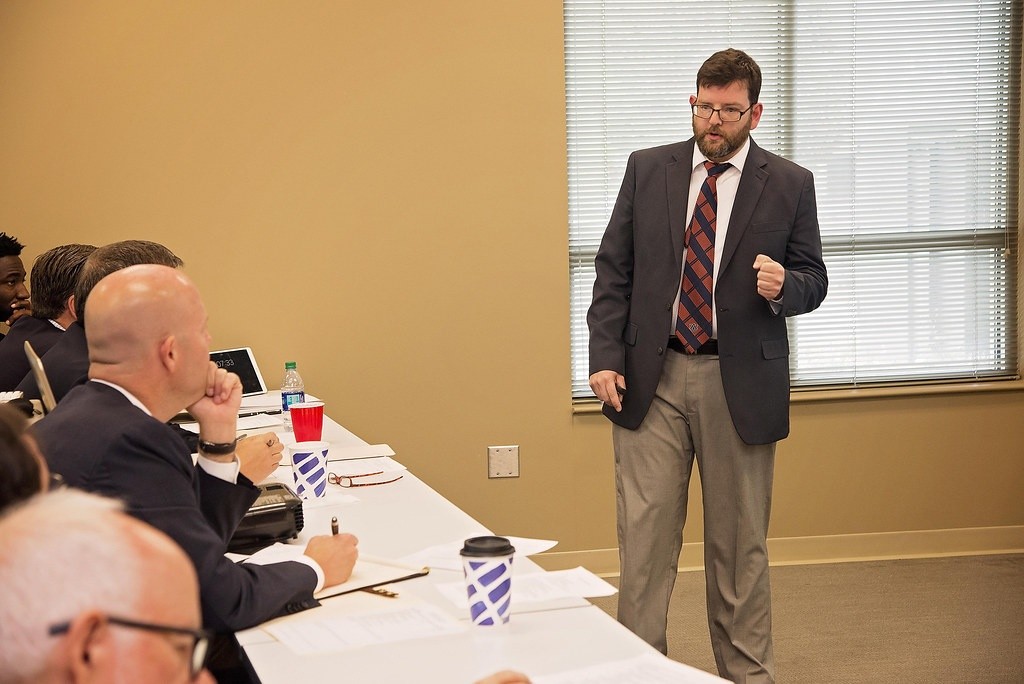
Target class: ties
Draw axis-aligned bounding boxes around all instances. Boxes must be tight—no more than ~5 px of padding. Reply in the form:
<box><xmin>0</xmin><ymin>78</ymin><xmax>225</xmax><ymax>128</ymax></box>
<box><xmin>674</xmin><ymin>160</ymin><xmax>733</xmax><ymax>353</ymax></box>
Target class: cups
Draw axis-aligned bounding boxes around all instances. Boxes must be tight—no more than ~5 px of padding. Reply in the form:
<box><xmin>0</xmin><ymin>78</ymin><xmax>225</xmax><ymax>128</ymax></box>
<box><xmin>458</xmin><ymin>537</ymin><xmax>515</xmax><ymax>627</ymax></box>
<box><xmin>289</xmin><ymin>403</ymin><xmax>325</xmax><ymax>443</ymax></box>
<box><xmin>288</xmin><ymin>442</ymin><xmax>330</xmax><ymax>501</ymax></box>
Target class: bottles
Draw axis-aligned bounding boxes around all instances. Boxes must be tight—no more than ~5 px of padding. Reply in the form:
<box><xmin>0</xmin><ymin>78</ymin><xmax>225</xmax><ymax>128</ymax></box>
<box><xmin>280</xmin><ymin>361</ymin><xmax>305</xmax><ymax>433</ymax></box>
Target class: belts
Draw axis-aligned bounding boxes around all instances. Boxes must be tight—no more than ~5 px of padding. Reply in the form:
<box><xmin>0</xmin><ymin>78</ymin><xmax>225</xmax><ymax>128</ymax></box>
<box><xmin>667</xmin><ymin>337</ymin><xmax>719</xmax><ymax>355</ymax></box>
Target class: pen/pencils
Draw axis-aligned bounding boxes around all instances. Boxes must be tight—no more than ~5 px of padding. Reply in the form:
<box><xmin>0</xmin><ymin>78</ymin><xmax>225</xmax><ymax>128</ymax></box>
<box><xmin>331</xmin><ymin>516</ymin><xmax>339</xmax><ymax>535</ymax></box>
<box><xmin>268</xmin><ymin>440</ymin><xmax>274</xmax><ymax>446</ymax></box>
<box><xmin>237</xmin><ymin>411</ymin><xmax>266</xmax><ymax>418</ymax></box>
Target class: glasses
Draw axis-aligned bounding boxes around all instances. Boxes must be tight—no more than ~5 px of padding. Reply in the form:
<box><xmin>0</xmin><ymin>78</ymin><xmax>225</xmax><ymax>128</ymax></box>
<box><xmin>48</xmin><ymin>615</ymin><xmax>211</xmax><ymax>680</ymax></box>
<box><xmin>327</xmin><ymin>470</ymin><xmax>403</xmax><ymax>488</ymax></box>
<box><xmin>691</xmin><ymin>99</ymin><xmax>753</xmax><ymax>122</ymax></box>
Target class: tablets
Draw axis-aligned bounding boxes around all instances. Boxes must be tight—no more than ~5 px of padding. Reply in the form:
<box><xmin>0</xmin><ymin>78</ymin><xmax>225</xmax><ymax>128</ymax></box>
<box><xmin>209</xmin><ymin>347</ymin><xmax>268</xmax><ymax>397</ymax></box>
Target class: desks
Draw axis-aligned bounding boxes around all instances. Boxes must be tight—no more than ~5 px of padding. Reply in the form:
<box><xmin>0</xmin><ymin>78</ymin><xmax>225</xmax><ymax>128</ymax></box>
<box><xmin>179</xmin><ymin>391</ymin><xmax>689</xmax><ymax>684</ymax></box>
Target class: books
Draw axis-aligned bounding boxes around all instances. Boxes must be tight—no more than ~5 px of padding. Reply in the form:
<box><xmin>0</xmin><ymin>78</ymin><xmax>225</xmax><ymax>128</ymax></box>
<box><xmin>240</xmin><ymin>543</ymin><xmax>429</xmax><ymax>599</ymax></box>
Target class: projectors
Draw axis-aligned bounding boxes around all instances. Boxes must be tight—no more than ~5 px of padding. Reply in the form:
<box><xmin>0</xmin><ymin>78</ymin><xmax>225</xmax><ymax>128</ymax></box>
<box><xmin>226</xmin><ymin>483</ymin><xmax>305</xmax><ymax>551</ymax></box>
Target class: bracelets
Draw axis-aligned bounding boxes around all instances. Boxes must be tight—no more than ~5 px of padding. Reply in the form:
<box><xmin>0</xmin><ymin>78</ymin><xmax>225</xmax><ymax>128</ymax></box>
<box><xmin>198</xmin><ymin>437</ymin><xmax>237</xmax><ymax>455</ymax></box>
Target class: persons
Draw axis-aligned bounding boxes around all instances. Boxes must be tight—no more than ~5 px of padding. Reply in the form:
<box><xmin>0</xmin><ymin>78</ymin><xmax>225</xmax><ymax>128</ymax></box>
<box><xmin>0</xmin><ymin>486</ymin><xmax>216</xmax><ymax>684</ymax></box>
<box><xmin>0</xmin><ymin>233</ymin><xmax>100</xmax><ymax>392</ymax></box>
<box><xmin>585</xmin><ymin>50</ymin><xmax>828</xmax><ymax>684</ymax></box>
<box><xmin>0</xmin><ymin>405</ymin><xmax>64</xmax><ymax>517</ymax></box>
<box><xmin>13</xmin><ymin>239</ymin><xmax>284</xmax><ymax>521</ymax></box>
<box><xmin>28</xmin><ymin>264</ymin><xmax>358</xmax><ymax>634</ymax></box>
